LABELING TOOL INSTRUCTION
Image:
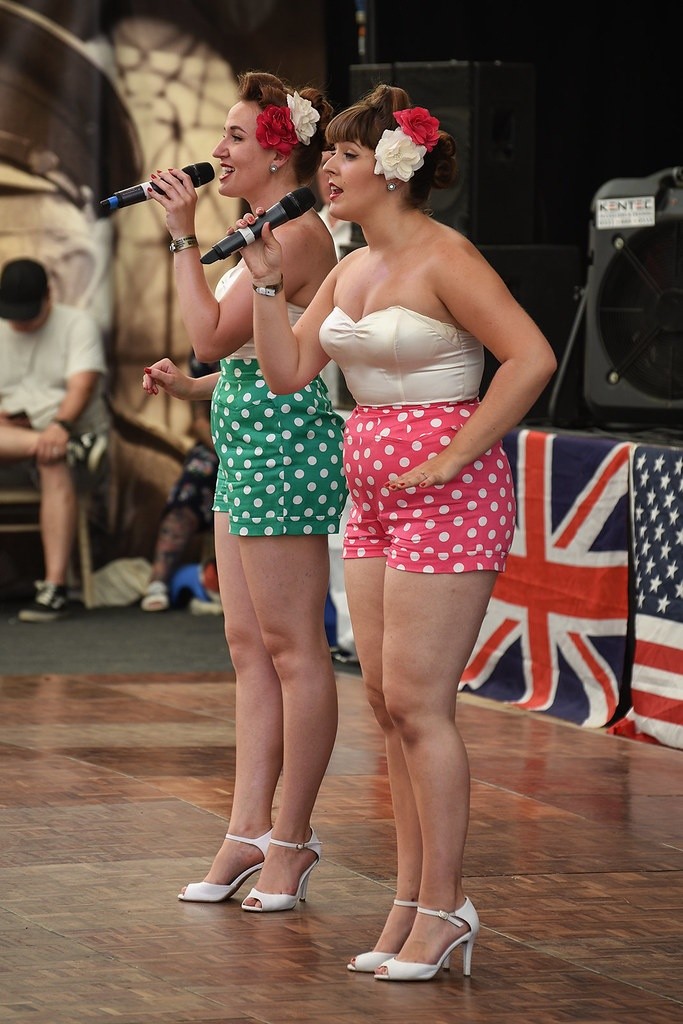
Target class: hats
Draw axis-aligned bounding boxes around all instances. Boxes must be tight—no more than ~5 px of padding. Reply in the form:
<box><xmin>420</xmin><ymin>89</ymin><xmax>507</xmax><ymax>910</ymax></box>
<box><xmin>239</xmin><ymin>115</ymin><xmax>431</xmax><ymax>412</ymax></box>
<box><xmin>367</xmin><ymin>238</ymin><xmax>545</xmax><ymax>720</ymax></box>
<box><xmin>0</xmin><ymin>259</ymin><xmax>47</xmax><ymax>321</ymax></box>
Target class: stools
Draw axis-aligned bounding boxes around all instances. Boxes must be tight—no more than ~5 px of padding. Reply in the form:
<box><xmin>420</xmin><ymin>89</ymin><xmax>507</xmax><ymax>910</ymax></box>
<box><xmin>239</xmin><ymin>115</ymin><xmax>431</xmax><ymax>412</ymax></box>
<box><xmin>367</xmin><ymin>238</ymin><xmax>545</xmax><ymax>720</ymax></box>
<box><xmin>0</xmin><ymin>425</ymin><xmax>120</xmax><ymax>613</ymax></box>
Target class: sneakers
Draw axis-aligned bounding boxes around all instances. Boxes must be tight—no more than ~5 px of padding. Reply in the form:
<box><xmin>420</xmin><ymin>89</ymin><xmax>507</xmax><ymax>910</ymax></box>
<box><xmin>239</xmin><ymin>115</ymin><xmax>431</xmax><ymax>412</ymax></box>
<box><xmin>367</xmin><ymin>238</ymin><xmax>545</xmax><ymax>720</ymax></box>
<box><xmin>63</xmin><ymin>432</ymin><xmax>108</xmax><ymax>475</ymax></box>
<box><xmin>18</xmin><ymin>579</ymin><xmax>74</xmax><ymax>622</ymax></box>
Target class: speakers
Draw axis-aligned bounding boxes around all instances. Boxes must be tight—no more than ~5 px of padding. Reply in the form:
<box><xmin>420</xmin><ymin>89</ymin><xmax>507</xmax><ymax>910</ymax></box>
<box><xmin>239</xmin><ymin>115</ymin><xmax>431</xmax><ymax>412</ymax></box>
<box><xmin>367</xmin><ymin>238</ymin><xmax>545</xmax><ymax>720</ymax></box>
<box><xmin>348</xmin><ymin>61</ymin><xmax>537</xmax><ymax>400</ymax></box>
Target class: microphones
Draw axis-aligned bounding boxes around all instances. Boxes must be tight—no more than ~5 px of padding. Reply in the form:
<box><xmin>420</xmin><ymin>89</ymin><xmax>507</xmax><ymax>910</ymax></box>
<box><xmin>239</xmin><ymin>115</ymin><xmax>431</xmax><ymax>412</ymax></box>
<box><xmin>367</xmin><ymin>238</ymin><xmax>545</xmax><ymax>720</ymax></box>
<box><xmin>199</xmin><ymin>186</ymin><xmax>316</xmax><ymax>264</ymax></box>
<box><xmin>99</xmin><ymin>162</ymin><xmax>215</xmax><ymax>210</ymax></box>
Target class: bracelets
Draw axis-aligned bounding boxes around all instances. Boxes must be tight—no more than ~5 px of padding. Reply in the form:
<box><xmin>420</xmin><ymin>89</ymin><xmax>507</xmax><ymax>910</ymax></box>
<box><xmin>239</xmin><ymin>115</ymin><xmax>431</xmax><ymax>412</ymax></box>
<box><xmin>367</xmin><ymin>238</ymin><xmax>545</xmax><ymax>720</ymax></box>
<box><xmin>251</xmin><ymin>273</ymin><xmax>284</xmax><ymax>297</ymax></box>
<box><xmin>169</xmin><ymin>235</ymin><xmax>200</xmax><ymax>252</ymax></box>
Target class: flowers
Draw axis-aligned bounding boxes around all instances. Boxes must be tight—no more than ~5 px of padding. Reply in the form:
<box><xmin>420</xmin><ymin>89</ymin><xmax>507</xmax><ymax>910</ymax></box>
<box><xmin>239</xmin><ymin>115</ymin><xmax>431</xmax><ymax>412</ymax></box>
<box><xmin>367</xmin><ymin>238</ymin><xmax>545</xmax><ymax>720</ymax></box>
<box><xmin>373</xmin><ymin>106</ymin><xmax>441</xmax><ymax>181</ymax></box>
<box><xmin>257</xmin><ymin>90</ymin><xmax>320</xmax><ymax>156</ymax></box>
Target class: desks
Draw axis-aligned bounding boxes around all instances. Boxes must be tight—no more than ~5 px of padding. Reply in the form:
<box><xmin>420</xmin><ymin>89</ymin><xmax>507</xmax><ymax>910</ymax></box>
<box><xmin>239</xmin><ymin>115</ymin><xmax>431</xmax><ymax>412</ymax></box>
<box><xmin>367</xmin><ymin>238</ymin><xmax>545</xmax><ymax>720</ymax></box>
<box><xmin>319</xmin><ymin>403</ymin><xmax>683</xmax><ymax>749</ymax></box>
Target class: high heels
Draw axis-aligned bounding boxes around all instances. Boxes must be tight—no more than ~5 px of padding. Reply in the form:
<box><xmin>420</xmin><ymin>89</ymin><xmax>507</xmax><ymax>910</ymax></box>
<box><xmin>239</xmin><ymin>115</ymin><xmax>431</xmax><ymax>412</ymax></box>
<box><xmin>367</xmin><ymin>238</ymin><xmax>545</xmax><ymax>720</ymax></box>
<box><xmin>241</xmin><ymin>827</ymin><xmax>322</xmax><ymax>912</ymax></box>
<box><xmin>176</xmin><ymin>826</ymin><xmax>273</xmax><ymax>903</ymax></box>
<box><xmin>373</xmin><ymin>895</ymin><xmax>480</xmax><ymax>982</ymax></box>
<box><xmin>345</xmin><ymin>897</ymin><xmax>451</xmax><ymax>974</ymax></box>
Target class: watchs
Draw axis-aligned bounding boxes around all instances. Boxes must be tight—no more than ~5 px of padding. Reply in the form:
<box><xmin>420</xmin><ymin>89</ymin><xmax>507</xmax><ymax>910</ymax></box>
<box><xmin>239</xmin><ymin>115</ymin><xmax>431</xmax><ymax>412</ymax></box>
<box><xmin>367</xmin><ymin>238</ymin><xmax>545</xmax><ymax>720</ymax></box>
<box><xmin>50</xmin><ymin>417</ymin><xmax>73</xmax><ymax>434</ymax></box>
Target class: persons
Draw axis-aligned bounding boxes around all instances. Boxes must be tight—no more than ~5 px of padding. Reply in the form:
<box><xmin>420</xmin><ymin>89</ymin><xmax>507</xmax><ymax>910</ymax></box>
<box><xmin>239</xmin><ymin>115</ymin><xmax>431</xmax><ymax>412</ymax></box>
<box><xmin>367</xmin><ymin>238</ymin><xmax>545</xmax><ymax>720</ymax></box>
<box><xmin>232</xmin><ymin>84</ymin><xmax>559</xmax><ymax>984</ymax></box>
<box><xmin>1</xmin><ymin>257</ymin><xmax>113</xmax><ymax>620</ymax></box>
<box><xmin>140</xmin><ymin>73</ymin><xmax>350</xmax><ymax>909</ymax></box>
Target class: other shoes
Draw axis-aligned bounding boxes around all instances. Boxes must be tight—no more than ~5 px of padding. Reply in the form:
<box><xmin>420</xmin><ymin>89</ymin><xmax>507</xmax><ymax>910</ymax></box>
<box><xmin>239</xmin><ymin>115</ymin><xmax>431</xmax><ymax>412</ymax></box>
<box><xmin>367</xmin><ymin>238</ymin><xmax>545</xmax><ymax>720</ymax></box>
<box><xmin>140</xmin><ymin>581</ymin><xmax>168</xmax><ymax>612</ymax></box>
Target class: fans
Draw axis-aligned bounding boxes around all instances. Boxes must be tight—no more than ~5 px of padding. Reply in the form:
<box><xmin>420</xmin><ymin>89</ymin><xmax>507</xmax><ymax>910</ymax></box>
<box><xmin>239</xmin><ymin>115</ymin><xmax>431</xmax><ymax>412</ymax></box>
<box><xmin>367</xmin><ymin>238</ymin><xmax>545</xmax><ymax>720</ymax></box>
<box><xmin>546</xmin><ymin>168</ymin><xmax>683</xmax><ymax>428</ymax></box>
